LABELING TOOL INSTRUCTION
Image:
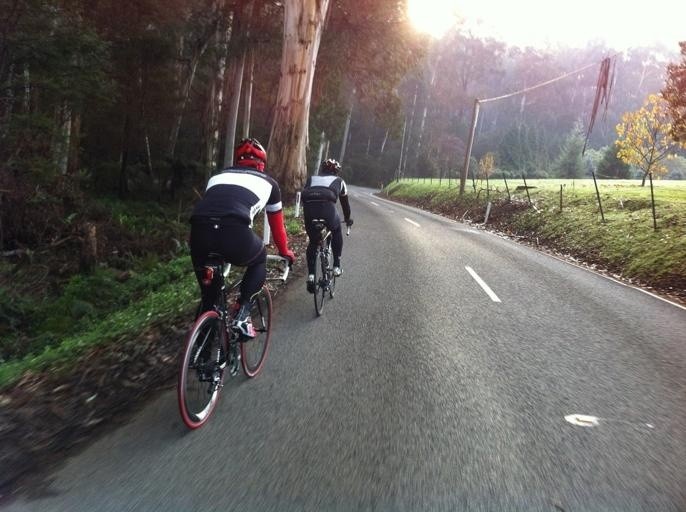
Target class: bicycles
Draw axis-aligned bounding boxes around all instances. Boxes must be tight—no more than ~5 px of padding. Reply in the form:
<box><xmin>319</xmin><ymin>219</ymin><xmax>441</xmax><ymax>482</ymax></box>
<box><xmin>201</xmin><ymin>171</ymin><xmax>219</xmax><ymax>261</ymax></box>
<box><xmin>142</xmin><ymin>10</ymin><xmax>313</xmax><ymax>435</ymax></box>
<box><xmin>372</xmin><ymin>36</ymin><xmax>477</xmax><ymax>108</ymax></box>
<box><xmin>178</xmin><ymin>219</ymin><xmax>350</xmax><ymax>429</ymax></box>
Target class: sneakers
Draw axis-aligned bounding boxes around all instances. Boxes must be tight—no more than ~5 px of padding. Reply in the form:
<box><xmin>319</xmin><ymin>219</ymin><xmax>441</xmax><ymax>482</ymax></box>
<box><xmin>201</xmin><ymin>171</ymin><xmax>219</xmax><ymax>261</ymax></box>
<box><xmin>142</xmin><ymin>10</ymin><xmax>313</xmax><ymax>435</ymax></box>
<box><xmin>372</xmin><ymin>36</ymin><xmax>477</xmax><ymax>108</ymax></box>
<box><xmin>306</xmin><ymin>274</ymin><xmax>316</xmax><ymax>294</ymax></box>
<box><xmin>333</xmin><ymin>267</ymin><xmax>344</xmax><ymax>277</ymax></box>
<box><xmin>232</xmin><ymin>317</ymin><xmax>258</xmax><ymax>338</ymax></box>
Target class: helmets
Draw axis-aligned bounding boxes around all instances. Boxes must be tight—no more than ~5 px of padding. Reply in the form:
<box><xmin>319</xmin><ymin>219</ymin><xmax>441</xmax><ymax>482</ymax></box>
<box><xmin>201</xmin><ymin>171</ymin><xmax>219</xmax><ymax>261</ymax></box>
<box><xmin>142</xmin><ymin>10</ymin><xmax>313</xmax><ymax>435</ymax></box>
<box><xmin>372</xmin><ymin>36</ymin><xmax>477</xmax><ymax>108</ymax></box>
<box><xmin>233</xmin><ymin>137</ymin><xmax>268</xmax><ymax>172</ymax></box>
<box><xmin>321</xmin><ymin>158</ymin><xmax>342</xmax><ymax>175</ymax></box>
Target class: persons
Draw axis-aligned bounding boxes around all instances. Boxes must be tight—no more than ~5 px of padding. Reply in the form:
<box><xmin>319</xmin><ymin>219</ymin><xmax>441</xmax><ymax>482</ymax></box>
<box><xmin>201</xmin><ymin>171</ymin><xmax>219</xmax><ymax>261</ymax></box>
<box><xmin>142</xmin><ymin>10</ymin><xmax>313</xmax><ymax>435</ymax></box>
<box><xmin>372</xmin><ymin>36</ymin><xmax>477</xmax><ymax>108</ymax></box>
<box><xmin>301</xmin><ymin>158</ymin><xmax>353</xmax><ymax>293</ymax></box>
<box><xmin>189</xmin><ymin>138</ymin><xmax>295</xmax><ymax>364</ymax></box>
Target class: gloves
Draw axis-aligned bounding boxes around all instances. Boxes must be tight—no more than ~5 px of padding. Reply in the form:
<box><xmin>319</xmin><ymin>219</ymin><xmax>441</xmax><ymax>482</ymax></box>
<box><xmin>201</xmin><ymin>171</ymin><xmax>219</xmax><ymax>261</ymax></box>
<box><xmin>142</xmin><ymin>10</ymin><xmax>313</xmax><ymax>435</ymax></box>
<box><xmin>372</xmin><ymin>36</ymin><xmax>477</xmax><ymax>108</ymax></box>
<box><xmin>346</xmin><ymin>220</ymin><xmax>353</xmax><ymax>226</ymax></box>
<box><xmin>280</xmin><ymin>249</ymin><xmax>296</xmax><ymax>265</ymax></box>
